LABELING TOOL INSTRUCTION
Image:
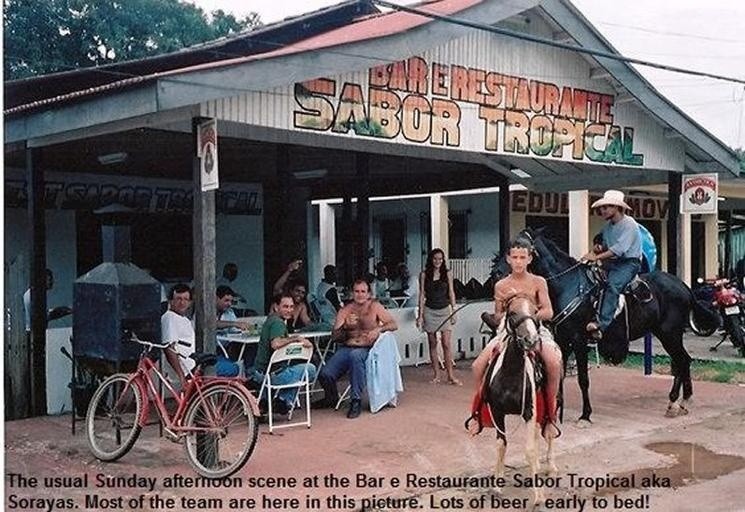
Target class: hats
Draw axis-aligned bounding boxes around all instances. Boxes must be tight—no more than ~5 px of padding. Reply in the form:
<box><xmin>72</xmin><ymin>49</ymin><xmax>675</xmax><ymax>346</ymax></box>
<box><xmin>591</xmin><ymin>190</ymin><xmax>633</xmax><ymax>211</ymax></box>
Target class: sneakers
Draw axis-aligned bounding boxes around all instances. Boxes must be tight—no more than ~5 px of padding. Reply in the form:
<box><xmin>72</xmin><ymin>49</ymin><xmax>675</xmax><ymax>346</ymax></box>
<box><xmin>347</xmin><ymin>398</ymin><xmax>361</xmax><ymax>418</ymax></box>
<box><xmin>587</xmin><ymin>322</ymin><xmax>603</xmax><ymax>341</ymax></box>
<box><xmin>272</xmin><ymin>398</ymin><xmax>289</xmax><ymax>415</ymax></box>
<box><xmin>260</xmin><ymin>401</ymin><xmax>268</xmax><ymax>415</ymax></box>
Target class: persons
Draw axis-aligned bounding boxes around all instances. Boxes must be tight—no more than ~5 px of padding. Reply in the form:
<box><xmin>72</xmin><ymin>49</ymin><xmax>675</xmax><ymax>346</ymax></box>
<box><xmin>271</xmin><ymin>260</ymin><xmax>312</xmax><ymax>327</ymax></box>
<box><xmin>216</xmin><ymin>262</ymin><xmax>247</xmax><ymax>303</ymax></box>
<box><xmin>251</xmin><ymin>293</ymin><xmax>316</xmax><ymax>413</ymax></box>
<box><xmin>23</xmin><ymin>269</ymin><xmax>55</xmax><ymax>332</ymax></box>
<box><xmin>467</xmin><ymin>237</ymin><xmax>563</xmax><ymax>443</ymax></box>
<box><xmin>212</xmin><ymin>285</ymin><xmax>253</xmax><ymax>334</ymax></box>
<box><xmin>317</xmin><ymin>278</ymin><xmax>397</xmax><ymax>417</ymax></box>
<box><xmin>160</xmin><ymin>284</ymin><xmax>240</xmax><ymax>384</ymax></box>
<box><xmin>414</xmin><ymin>248</ymin><xmax>464</xmax><ymax>386</ymax></box>
<box><xmin>315</xmin><ymin>259</ymin><xmax>420</xmax><ymax>324</ymax></box>
<box><xmin>583</xmin><ymin>189</ymin><xmax>641</xmax><ymax>340</ymax></box>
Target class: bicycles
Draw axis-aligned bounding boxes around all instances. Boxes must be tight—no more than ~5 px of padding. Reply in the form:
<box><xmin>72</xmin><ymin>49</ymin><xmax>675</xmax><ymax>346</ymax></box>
<box><xmin>83</xmin><ymin>330</ymin><xmax>262</xmax><ymax>481</ymax></box>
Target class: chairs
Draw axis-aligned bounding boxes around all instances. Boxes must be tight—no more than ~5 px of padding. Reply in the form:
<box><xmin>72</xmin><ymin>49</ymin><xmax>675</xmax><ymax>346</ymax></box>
<box><xmin>163</xmin><ymin>285</ymin><xmax>408</xmax><ymax>436</ymax></box>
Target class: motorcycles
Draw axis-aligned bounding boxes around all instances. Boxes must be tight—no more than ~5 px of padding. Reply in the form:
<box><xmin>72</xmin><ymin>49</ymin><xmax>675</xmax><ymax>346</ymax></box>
<box><xmin>687</xmin><ymin>283</ymin><xmax>721</xmax><ymax>338</ymax></box>
<box><xmin>696</xmin><ymin>259</ymin><xmax>744</xmax><ymax>358</ymax></box>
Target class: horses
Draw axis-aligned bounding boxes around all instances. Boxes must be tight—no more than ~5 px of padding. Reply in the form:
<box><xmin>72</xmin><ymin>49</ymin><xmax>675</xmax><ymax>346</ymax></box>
<box><xmin>487</xmin><ymin>225</ymin><xmax>714</xmax><ymax>430</ymax></box>
<box><xmin>484</xmin><ymin>287</ymin><xmax>563</xmax><ymax>512</ymax></box>
